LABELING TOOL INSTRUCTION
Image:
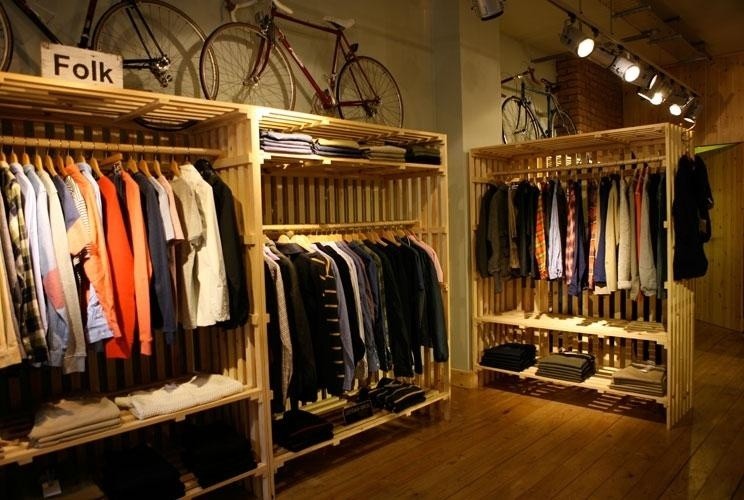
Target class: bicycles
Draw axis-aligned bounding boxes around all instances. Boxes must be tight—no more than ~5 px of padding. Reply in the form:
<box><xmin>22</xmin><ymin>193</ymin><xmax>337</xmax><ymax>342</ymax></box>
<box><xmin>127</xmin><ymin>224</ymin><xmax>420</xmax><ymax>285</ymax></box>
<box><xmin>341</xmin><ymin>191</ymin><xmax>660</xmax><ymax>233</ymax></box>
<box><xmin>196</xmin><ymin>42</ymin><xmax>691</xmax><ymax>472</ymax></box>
<box><xmin>500</xmin><ymin>61</ymin><xmax>577</xmax><ymax>145</ymax></box>
<box><xmin>199</xmin><ymin>1</ymin><xmax>404</xmax><ymax>146</ymax></box>
<box><xmin>0</xmin><ymin>1</ymin><xmax>219</xmax><ymax>131</ymax></box>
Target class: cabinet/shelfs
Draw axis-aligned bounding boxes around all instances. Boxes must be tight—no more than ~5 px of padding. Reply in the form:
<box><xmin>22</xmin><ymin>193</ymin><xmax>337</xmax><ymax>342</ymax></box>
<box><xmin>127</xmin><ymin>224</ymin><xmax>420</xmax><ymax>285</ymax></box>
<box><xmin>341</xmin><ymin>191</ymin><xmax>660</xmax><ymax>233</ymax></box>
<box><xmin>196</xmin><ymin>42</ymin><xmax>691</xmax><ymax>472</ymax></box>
<box><xmin>0</xmin><ymin>375</ymin><xmax>267</xmax><ymax>500</ymax></box>
<box><xmin>472</xmin><ymin>309</ymin><xmax>693</xmax><ymax>429</ymax></box>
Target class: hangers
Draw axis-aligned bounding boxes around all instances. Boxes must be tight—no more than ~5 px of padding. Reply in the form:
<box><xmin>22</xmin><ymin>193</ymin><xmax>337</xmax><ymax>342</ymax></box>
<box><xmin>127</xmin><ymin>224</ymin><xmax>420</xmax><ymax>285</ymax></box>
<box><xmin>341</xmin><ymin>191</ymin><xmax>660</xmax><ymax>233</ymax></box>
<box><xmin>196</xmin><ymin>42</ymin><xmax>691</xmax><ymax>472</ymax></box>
<box><xmin>491</xmin><ymin>154</ymin><xmax>665</xmax><ymax>190</ymax></box>
<box><xmin>0</xmin><ymin>136</ymin><xmax>213</xmax><ymax>179</ymax></box>
<box><xmin>263</xmin><ymin>220</ymin><xmax>417</xmax><ymax>250</ymax></box>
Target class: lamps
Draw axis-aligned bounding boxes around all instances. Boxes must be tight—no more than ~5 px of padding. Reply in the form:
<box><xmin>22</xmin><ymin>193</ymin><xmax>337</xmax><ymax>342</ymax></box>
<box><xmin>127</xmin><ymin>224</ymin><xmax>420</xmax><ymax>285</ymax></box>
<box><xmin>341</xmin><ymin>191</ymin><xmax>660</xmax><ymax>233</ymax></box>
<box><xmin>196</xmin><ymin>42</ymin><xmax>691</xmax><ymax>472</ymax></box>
<box><xmin>558</xmin><ymin>14</ymin><xmax>702</xmax><ymax>125</ymax></box>
<box><xmin>469</xmin><ymin>0</ymin><xmax>504</xmax><ymax>20</ymax></box>
